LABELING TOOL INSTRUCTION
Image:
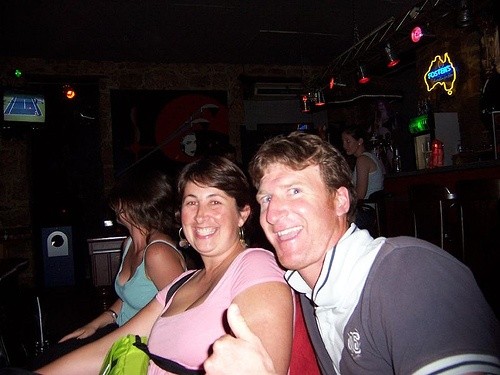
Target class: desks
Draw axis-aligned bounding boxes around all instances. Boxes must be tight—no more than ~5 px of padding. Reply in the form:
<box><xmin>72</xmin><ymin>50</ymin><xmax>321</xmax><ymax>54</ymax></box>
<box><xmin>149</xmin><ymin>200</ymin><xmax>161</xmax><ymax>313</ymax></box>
<box><xmin>86</xmin><ymin>234</ymin><xmax>127</xmax><ymax>310</ymax></box>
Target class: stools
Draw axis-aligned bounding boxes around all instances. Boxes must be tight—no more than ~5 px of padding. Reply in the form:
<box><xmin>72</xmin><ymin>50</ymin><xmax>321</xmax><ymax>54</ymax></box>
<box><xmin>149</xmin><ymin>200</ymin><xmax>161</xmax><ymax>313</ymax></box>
<box><xmin>407</xmin><ymin>183</ymin><xmax>465</xmax><ymax>260</ymax></box>
<box><xmin>362</xmin><ymin>189</ymin><xmax>387</xmax><ymax>237</ymax></box>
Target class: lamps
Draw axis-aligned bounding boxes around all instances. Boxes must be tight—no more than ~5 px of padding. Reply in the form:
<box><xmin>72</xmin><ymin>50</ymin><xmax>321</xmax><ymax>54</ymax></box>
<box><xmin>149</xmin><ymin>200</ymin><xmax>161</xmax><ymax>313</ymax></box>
<box><xmin>63</xmin><ymin>82</ymin><xmax>79</xmax><ymax>100</ymax></box>
<box><xmin>301</xmin><ymin>7</ymin><xmax>471</xmax><ymax>113</ymax></box>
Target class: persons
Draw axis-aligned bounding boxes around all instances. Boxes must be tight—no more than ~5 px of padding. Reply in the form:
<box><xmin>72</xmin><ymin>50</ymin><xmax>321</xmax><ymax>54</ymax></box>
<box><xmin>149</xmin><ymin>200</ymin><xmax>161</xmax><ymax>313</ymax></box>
<box><xmin>36</xmin><ymin>162</ymin><xmax>188</xmax><ymax>369</ymax></box>
<box><xmin>203</xmin><ymin>131</ymin><xmax>500</xmax><ymax>375</ymax></box>
<box><xmin>341</xmin><ymin>126</ymin><xmax>385</xmax><ymax>228</ymax></box>
<box><xmin>32</xmin><ymin>154</ymin><xmax>295</xmax><ymax>374</ymax></box>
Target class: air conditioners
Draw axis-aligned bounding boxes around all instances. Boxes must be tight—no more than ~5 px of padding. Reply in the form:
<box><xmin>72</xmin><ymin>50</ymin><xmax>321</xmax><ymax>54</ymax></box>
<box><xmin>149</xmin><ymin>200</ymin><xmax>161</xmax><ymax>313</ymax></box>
<box><xmin>243</xmin><ymin>76</ymin><xmax>305</xmax><ymax>101</ymax></box>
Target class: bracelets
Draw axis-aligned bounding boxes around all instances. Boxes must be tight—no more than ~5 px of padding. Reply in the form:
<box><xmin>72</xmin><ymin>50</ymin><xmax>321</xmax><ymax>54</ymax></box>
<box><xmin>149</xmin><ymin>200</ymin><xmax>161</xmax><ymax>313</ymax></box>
<box><xmin>104</xmin><ymin>308</ymin><xmax>118</xmax><ymax>323</ymax></box>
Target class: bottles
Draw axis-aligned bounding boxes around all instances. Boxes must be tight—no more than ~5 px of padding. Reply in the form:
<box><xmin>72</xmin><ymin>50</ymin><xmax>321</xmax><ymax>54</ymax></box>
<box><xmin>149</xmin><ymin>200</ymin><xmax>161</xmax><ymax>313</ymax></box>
<box><xmin>430</xmin><ymin>139</ymin><xmax>445</xmax><ymax>166</ymax></box>
<box><xmin>370</xmin><ymin>133</ymin><xmax>402</xmax><ymax>174</ymax></box>
